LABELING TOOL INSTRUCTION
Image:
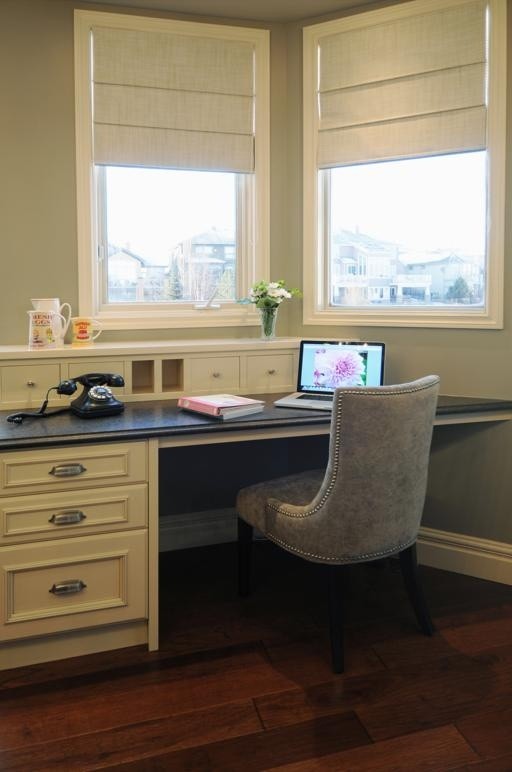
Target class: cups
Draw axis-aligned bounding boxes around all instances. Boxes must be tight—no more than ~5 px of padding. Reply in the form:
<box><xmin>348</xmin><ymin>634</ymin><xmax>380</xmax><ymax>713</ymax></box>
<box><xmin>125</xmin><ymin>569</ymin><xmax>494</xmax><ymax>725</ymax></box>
<box><xmin>27</xmin><ymin>298</ymin><xmax>103</xmax><ymax>350</ymax></box>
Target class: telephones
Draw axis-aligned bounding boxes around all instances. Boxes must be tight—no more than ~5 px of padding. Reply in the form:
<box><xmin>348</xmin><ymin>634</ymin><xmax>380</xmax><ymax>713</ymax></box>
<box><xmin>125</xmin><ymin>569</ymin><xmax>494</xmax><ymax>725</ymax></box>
<box><xmin>57</xmin><ymin>373</ymin><xmax>125</xmax><ymax>420</ymax></box>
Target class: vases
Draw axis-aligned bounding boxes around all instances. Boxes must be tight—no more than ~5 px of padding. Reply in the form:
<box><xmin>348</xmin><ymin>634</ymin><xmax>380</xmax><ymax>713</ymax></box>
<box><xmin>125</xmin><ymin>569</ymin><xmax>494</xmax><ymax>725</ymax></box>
<box><xmin>254</xmin><ymin>307</ymin><xmax>278</xmax><ymax>342</ymax></box>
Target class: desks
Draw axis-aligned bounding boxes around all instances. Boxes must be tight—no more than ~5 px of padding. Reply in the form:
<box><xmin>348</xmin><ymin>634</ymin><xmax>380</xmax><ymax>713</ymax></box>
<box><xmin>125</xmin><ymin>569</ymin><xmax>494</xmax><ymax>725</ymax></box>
<box><xmin>0</xmin><ymin>335</ymin><xmax>512</xmax><ymax>673</ymax></box>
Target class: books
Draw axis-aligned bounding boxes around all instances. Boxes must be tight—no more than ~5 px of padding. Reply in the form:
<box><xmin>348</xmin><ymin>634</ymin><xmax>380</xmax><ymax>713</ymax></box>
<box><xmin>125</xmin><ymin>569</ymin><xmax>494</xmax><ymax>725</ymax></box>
<box><xmin>178</xmin><ymin>406</ymin><xmax>265</xmax><ymax>420</ymax></box>
<box><xmin>176</xmin><ymin>393</ymin><xmax>266</xmax><ymax>417</ymax></box>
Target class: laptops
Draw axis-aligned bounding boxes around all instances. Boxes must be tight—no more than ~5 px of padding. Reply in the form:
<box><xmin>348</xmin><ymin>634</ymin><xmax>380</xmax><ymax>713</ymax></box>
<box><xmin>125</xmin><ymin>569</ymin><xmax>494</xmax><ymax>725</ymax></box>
<box><xmin>274</xmin><ymin>340</ymin><xmax>385</xmax><ymax>411</ymax></box>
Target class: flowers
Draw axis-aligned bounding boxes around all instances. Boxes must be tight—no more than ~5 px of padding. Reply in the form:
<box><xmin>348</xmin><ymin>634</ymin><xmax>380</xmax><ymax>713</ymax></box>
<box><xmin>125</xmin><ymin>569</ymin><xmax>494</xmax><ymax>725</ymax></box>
<box><xmin>235</xmin><ymin>280</ymin><xmax>303</xmax><ymax>335</ymax></box>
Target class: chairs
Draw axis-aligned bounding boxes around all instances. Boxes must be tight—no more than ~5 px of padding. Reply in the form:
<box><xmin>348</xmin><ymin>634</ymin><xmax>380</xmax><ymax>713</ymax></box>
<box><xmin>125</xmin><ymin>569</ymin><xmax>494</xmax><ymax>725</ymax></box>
<box><xmin>233</xmin><ymin>375</ymin><xmax>441</xmax><ymax>670</ymax></box>
